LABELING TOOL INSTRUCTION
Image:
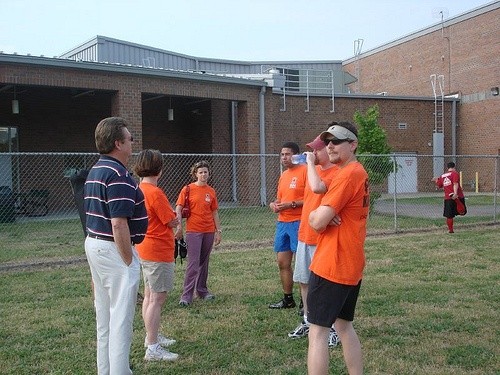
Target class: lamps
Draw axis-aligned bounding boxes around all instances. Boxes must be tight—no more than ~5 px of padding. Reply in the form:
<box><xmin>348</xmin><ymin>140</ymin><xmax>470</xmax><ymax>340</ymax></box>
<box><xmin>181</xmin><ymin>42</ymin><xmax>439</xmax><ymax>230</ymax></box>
<box><xmin>490</xmin><ymin>87</ymin><xmax>498</xmax><ymax>95</ymax></box>
<box><xmin>168</xmin><ymin>95</ymin><xmax>174</xmax><ymax>121</ymax></box>
<box><xmin>11</xmin><ymin>84</ymin><xmax>19</xmax><ymax>114</ymax></box>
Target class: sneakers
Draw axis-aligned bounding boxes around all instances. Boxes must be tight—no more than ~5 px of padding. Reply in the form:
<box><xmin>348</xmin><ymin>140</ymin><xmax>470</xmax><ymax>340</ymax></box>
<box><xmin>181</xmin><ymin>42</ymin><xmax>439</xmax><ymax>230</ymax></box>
<box><xmin>144</xmin><ymin>344</ymin><xmax>179</xmax><ymax>362</ymax></box>
<box><xmin>327</xmin><ymin>327</ymin><xmax>342</xmax><ymax>348</ymax></box>
<box><xmin>298</xmin><ymin>299</ymin><xmax>305</xmax><ymax>317</ymax></box>
<box><xmin>269</xmin><ymin>298</ymin><xmax>296</xmax><ymax>309</ymax></box>
<box><xmin>288</xmin><ymin>320</ymin><xmax>310</xmax><ymax>339</ymax></box>
<box><xmin>144</xmin><ymin>333</ymin><xmax>177</xmax><ymax>348</ymax></box>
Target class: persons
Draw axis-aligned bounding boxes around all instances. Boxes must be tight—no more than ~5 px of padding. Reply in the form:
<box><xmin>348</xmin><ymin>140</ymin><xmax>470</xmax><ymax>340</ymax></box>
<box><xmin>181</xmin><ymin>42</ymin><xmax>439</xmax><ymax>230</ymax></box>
<box><xmin>131</xmin><ymin>149</ymin><xmax>179</xmax><ymax>360</ymax></box>
<box><xmin>306</xmin><ymin>121</ymin><xmax>369</xmax><ymax>374</ymax></box>
<box><xmin>436</xmin><ymin>161</ymin><xmax>467</xmax><ymax>233</ymax></box>
<box><xmin>84</xmin><ymin>117</ymin><xmax>148</xmax><ymax>375</ymax></box>
<box><xmin>289</xmin><ymin>132</ymin><xmax>341</xmax><ymax>346</ymax></box>
<box><xmin>269</xmin><ymin>142</ymin><xmax>307</xmax><ymax>314</ymax></box>
<box><xmin>174</xmin><ymin>161</ymin><xmax>222</xmax><ymax>306</ymax></box>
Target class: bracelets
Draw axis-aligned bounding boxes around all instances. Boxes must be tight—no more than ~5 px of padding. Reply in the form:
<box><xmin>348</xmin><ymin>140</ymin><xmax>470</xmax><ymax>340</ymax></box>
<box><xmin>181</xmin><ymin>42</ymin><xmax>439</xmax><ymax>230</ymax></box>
<box><xmin>218</xmin><ymin>230</ymin><xmax>222</xmax><ymax>233</ymax></box>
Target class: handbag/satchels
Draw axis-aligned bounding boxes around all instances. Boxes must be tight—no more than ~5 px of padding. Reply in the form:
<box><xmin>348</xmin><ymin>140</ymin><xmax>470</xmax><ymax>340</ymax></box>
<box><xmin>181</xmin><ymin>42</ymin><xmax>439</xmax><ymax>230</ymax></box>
<box><xmin>180</xmin><ymin>186</ymin><xmax>190</xmax><ymax>218</ymax></box>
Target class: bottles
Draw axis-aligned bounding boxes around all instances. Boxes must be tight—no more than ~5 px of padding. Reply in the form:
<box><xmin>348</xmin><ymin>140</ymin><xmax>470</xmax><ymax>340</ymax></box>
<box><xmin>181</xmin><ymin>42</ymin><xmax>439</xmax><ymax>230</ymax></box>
<box><xmin>291</xmin><ymin>154</ymin><xmax>309</xmax><ymax>164</ymax></box>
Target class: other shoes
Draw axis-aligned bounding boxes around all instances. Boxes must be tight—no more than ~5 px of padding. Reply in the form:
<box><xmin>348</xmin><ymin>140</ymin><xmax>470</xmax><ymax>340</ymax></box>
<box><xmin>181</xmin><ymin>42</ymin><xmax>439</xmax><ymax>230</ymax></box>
<box><xmin>199</xmin><ymin>292</ymin><xmax>216</xmax><ymax>302</ymax></box>
<box><xmin>447</xmin><ymin>230</ymin><xmax>454</xmax><ymax>235</ymax></box>
<box><xmin>179</xmin><ymin>298</ymin><xmax>191</xmax><ymax>308</ymax></box>
<box><xmin>449</xmin><ymin>193</ymin><xmax>458</xmax><ymax>197</ymax></box>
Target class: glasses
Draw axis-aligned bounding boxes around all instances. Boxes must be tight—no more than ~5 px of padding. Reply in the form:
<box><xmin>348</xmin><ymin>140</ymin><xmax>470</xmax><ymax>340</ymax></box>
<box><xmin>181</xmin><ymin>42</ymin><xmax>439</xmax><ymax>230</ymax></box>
<box><xmin>322</xmin><ymin>137</ymin><xmax>348</xmax><ymax>145</ymax></box>
<box><xmin>121</xmin><ymin>136</ymin><xmax>133</xmax><ymax>142</ymax></box>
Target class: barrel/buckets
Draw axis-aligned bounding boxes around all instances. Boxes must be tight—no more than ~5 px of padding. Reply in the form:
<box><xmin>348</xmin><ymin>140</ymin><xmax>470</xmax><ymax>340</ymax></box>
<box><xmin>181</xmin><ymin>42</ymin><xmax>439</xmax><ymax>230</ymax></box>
<box><xmin>0</xmin><ymin>186</ymin><xmax>17</xmax><ymax>223</ymax></box>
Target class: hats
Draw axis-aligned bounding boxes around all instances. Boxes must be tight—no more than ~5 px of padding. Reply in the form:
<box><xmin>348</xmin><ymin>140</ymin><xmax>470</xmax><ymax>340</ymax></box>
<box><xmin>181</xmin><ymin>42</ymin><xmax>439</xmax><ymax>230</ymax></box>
<box><xmin>320</xmin><ymin>125</ymin><xmax>358</xmax><ymax>143</ymax></box>
<box><xmin>305</xmin><ymin>132</ymin><xmax>326</xmax><ymax>150</ymax></box>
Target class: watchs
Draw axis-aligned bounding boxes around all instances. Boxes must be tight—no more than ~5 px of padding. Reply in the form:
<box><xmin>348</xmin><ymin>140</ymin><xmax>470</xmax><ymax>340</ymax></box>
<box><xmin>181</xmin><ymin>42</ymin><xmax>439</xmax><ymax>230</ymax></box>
<box><xmin>291</xmin><ymin>201</ymin><xmax>296</xmax><ymax>209</ymax></box>
